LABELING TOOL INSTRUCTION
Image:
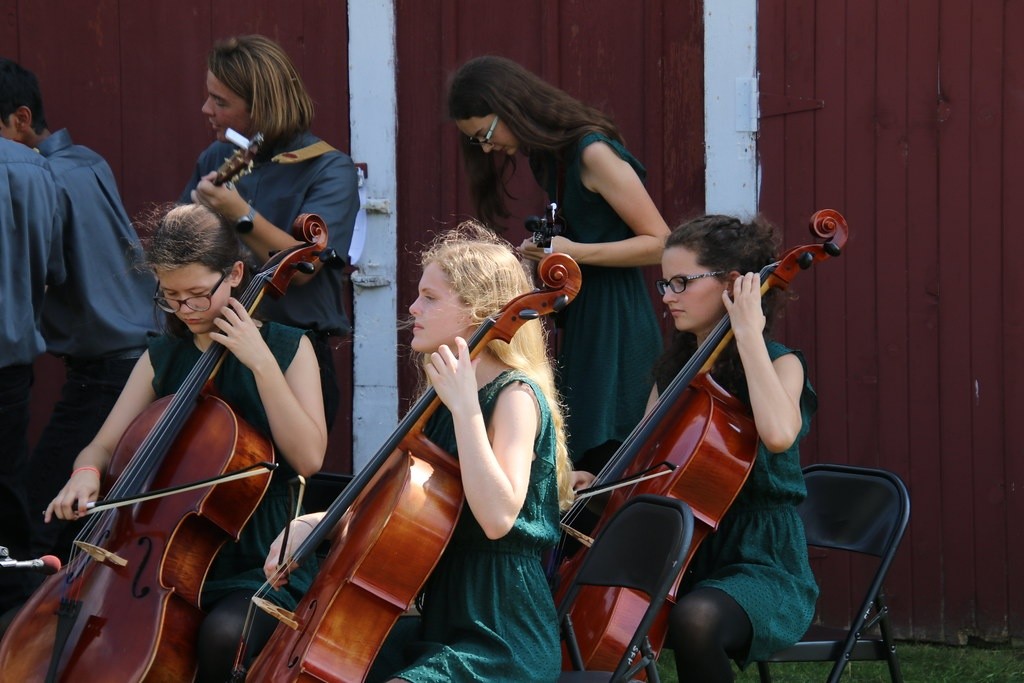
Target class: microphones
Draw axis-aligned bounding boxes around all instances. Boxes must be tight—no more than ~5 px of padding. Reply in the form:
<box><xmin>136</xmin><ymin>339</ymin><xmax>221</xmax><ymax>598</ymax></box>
<box><xmin>0</xmin><ymin>555</ymin><xmax>61</xmax><ymax>575</ymax></box>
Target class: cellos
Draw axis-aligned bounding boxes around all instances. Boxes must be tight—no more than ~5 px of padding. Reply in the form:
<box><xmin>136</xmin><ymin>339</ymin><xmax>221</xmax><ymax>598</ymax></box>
<box><xmin>0</xmin><ymin>207</ymin><xmax>850</xmax><ymax>683</ymax></box>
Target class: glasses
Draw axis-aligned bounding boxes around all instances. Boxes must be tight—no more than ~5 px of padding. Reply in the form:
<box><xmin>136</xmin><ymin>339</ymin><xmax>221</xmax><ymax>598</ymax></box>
<box><xmin>153</xmin><ymin>263</ymin><xmax>237</xmax><ymax>313</ymax></box>
<box><xmin>657</xmin><ymin>269</ymin><xmax>742</xmax><ymax>296</ymax></box>
<box><xmin>470</xmin><ymin>115</ymin><xmax>498</xmax><ymax>145</ymax></box>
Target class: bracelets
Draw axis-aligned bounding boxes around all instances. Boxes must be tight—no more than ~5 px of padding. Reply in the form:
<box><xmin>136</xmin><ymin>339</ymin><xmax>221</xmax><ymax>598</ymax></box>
<box><xmin>293</xmin><ymin>519</ymin><xmax>314</xmax><ymax>530</ymax></box>
<box><xmin>70</xmin><ymin>466</ymin><xmax>101</xmax><ymax>479</ymax></box>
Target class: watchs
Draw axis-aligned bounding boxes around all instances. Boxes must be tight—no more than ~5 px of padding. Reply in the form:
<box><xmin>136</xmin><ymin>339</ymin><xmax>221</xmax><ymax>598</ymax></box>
<box><xmin>228</xmin><ymin>205</ymin><xmax>255</xmax><ymax>234</ymax></box>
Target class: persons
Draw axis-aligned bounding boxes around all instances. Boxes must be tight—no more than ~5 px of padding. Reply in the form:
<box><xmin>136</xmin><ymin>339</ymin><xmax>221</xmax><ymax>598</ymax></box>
<box><xmin>177</xmin><ymin>34</ymin><xmax>360</xmax><ymax>516</ymax></box>
<box><xmin>263</xmin><ymin>220</ymin><xmax>576</xmax><ymax>683</ymax></box>
<box><xmin>45</xmin><ymin>205</ymin><xmax>328</xmax><ymax>683</ymax></box>
<box><xmin>572</xmin><ymin>215</ymin><xmax>819</xmax><ymax>683</ymax></box>
<box><xmin>0</xmin><ymin>56</ymin><xmax>170</xmax><ymax>638</ymax></box>
<box><xmin>447</xmin><ymin>54</ymin><xmax>672</xmax><ymax>478</ymax></box>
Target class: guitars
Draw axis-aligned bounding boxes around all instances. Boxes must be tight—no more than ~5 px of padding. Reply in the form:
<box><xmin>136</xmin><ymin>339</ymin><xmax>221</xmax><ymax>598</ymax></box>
<box><xmin>181</xmin><ymin>130</ymin><xmax>266</xmax><ymax>206</ymax></box>
<box><xmin>523</xmin><ymin>203</ymin><xmax>560</xmax><ymax>293</ymax></box>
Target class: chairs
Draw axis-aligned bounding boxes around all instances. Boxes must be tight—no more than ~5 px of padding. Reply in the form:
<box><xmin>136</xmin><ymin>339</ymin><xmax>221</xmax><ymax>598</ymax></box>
<box><xmin>754</xmin><ymin>463</ymin><xmax>909</xmax><ymax>683</ymax></box>
<box><xmin>292</xmin><ymin>471</ymin><xmax>355</xmax><ymax>513</ymax></box>
<box><xmin>557</xmin><ymin>494</ymin><xmax>693</xmax><ymax>683</ymax></box>
